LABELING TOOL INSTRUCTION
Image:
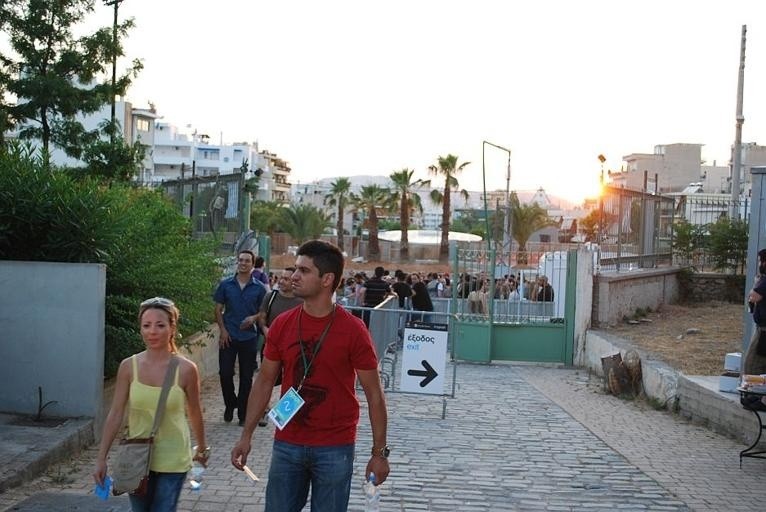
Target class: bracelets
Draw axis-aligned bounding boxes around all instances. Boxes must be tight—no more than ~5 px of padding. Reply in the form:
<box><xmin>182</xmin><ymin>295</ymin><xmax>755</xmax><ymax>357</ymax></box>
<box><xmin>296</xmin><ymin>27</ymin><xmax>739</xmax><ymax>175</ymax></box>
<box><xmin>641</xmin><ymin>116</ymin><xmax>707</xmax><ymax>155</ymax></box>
<box><xmin>197</xmin><ymin>447</ymin><xmax>211</xmax><ymax>459</ymax></box>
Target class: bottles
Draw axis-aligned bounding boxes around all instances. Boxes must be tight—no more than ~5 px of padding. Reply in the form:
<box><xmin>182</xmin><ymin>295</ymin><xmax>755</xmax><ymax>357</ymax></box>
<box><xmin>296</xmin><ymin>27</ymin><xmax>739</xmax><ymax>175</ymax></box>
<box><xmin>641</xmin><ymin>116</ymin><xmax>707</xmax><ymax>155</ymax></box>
<box><xmin>188</xmin><ymin>446</ymin><xmax>206</xmax><ymax>493</ymax></box>
<box><xmin>362</xmin><ymin>475</ymin><xmax>381</xmax><ymax>511</ymax></box>
<box><xmin>748</xmin><ymin>295</ymin><xmax>755</xmax><ymax>313</ymax></box>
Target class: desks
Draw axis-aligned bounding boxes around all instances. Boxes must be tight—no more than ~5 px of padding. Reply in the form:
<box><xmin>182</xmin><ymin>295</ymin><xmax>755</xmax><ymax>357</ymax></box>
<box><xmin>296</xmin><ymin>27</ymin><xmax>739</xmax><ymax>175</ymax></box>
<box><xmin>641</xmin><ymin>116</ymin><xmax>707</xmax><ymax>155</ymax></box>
<box><xmin>736</xmin><ymin>386</ymin><xmax>766</xmax><ymax>470</ymax></box>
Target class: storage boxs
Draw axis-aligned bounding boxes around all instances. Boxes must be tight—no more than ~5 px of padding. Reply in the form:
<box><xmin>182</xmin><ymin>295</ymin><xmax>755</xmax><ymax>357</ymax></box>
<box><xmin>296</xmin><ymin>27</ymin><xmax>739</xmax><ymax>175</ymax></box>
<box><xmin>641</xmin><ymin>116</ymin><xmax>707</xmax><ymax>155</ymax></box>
<box><xmin>719</xmin><ymin>373</ymin><xmax>741</xmax><ymax>394</ymax></box>
<box><xmin>724</xmin><ymin>353</ymin><xmax>742</xmax><ymax>371</ymax></box>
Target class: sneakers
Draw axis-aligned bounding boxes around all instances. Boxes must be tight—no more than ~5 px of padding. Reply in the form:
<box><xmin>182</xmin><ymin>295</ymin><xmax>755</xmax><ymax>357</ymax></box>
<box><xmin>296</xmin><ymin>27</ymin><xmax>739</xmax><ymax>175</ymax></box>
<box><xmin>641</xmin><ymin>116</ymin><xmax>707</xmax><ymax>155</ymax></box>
<box><xmin>224</xmin><ymin>401</ymin><xmax>271</xmax><ymax>428</ymax></box>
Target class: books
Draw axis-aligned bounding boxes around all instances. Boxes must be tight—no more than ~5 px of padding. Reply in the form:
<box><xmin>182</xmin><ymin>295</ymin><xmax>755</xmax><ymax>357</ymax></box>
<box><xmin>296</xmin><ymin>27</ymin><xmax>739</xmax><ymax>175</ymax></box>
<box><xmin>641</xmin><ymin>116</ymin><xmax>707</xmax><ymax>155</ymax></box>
<box><xmin>742</xmin><ymin>374</ymin><xmax>766</xmax><ymax>393</ymax></box>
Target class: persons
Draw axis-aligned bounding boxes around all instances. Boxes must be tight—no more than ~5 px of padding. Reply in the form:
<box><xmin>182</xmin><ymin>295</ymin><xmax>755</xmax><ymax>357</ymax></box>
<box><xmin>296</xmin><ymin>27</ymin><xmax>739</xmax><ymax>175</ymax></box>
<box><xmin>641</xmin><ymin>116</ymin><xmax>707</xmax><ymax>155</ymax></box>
<box><xmin>359</xmin><ymin>267</ymin><xmax>434</xmax><ymax>329</ymax></box>
<box><xmin>256</xmin><ymin>266</ymin><xmax>304</xmax><ymax>427</ymax></box>
<box><xmin>252</xmin><ymin>258</ymin><xmax>280</xmax><ymax>291</ymax></box>
<box><xmin>419</xmin><ymin>271</ymin><xmax>476</xmax><ymax>299</ymax></box>
<box><xmin>480</xmin><ymin>271</ymin><xmax>554</xmax><ymax>302</ymax></box>
<box><xmin>744</xmin><ymin>249</ymin><xmax>765</xmax><ymax>375</ymax></box>
<box><xmin>93</xmin><ymin>297</ymin><xmax>208</xmax><ymax>512</ymax></box>
<box><xmin>336</xmin><ymin>271</ymin><xmax>369</xmax><ymax>317</ymax></box>
<box><xmin>213</xmin><ymin>250</ymin><xmax>266</xmax><ymax>426</ymax></box>
<box><xmin>467</xmin><ymin>279</ymin><xmax>488</xmax><ymax>321</ymax></box>
<box><xmin>231</xmin><ymin>239</ymin><xmax>391</xmax><ymax>511</ymax></box>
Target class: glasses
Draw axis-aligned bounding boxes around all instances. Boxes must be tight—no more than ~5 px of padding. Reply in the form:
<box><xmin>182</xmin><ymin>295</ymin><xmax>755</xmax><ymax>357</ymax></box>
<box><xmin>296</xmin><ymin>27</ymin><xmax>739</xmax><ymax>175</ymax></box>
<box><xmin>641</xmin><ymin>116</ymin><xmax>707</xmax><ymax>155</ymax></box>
<box><xmin>141</xmin><ymin>297</ymin><xmax>174</xmax><ymax>308</ymax></box>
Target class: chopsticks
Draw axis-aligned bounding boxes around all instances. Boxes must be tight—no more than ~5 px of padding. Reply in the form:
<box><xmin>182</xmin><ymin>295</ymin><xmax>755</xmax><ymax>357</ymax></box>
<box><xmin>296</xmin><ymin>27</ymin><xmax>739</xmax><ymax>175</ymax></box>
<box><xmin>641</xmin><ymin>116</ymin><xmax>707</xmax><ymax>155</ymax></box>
<box><xmin>237</xmin><ymin>459</ymin><xmax>261</xmax><ymax>482</ymax></box>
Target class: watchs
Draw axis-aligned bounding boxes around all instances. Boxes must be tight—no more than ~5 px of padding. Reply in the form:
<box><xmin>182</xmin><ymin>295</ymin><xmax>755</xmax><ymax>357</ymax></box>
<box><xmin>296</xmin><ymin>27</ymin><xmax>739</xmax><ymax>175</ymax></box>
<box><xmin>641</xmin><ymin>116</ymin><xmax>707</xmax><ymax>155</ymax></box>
<box><xmin>371</xmin><ymin>445</ymin><xmax>390</xmax><ymax>457</ymax></box>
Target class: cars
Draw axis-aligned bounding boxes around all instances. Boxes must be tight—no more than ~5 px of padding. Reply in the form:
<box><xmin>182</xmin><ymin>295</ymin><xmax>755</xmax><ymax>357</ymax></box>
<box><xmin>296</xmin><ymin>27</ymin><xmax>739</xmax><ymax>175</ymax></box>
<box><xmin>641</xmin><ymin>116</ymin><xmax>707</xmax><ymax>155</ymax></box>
<box><xmin>519</xmin><ymin>269</ymin><xmax>540</xmax><ymax>283</ymax></box>
<box><xmin>427</xmin><ymin>279</ymin><xmax>451</xmax><ymax>296</ymax></box>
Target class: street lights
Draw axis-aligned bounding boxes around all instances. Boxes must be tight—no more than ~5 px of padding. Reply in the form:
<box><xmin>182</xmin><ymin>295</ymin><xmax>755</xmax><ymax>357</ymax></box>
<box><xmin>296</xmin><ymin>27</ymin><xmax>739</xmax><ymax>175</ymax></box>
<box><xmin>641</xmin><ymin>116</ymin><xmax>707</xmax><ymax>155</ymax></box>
<box><xmin>597</xmin><ymin>154</ymin><xmax>606</xmax><ymax>271</ymax></box>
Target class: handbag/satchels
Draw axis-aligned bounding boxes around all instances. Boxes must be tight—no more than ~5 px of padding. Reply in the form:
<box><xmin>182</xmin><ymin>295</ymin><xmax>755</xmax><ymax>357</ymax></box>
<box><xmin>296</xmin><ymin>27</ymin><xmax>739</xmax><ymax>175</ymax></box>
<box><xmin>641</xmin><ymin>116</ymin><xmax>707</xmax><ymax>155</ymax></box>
<box><xmin>111</xmin><ymin>439</ymin><xmax>153</xmax><ymax>496</ymax></box>
